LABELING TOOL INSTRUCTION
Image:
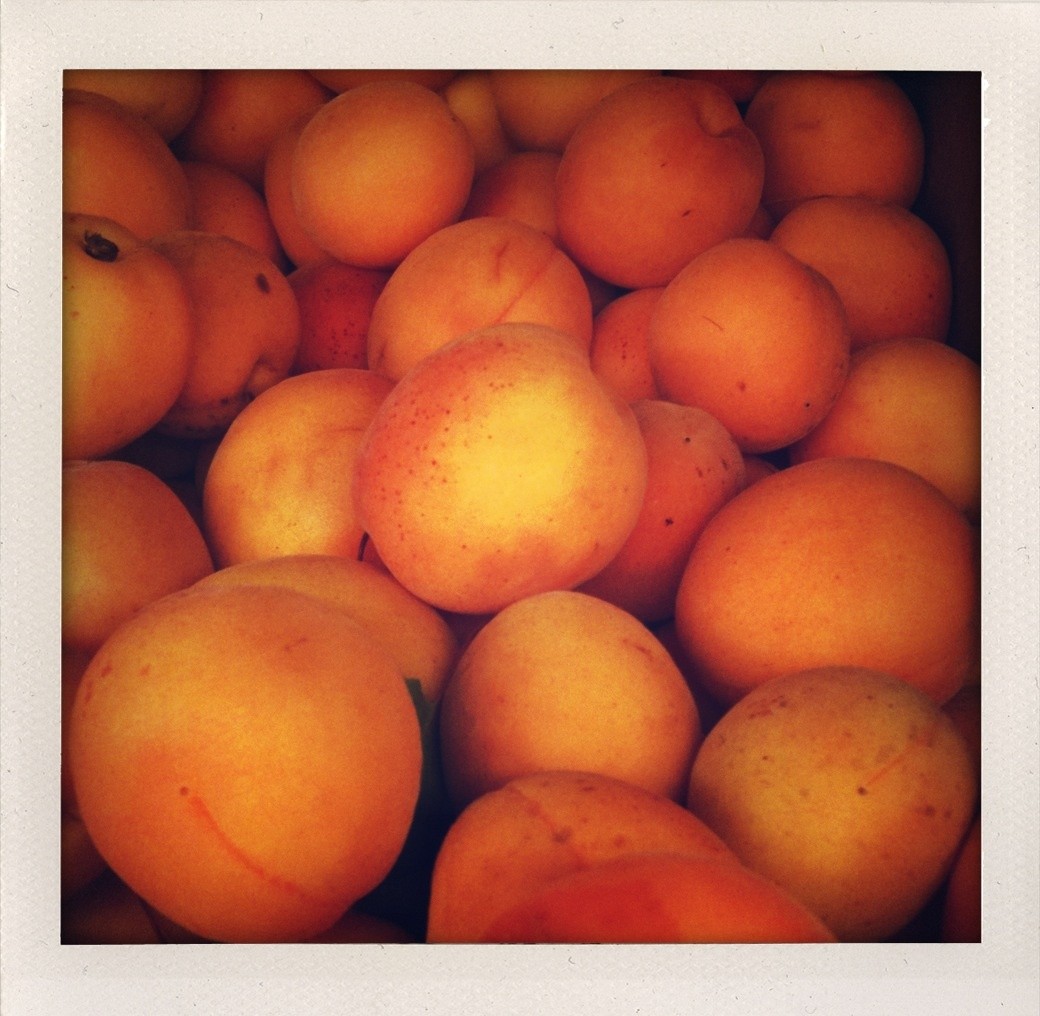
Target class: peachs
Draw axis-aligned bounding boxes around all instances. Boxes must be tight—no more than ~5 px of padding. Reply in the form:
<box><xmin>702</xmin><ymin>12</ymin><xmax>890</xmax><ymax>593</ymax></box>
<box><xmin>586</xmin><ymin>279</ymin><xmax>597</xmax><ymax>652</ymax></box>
<box><xmin>62</xmin><ymin>68</ymin><xmax>981</xmax><ymax>944</ymax></box>
